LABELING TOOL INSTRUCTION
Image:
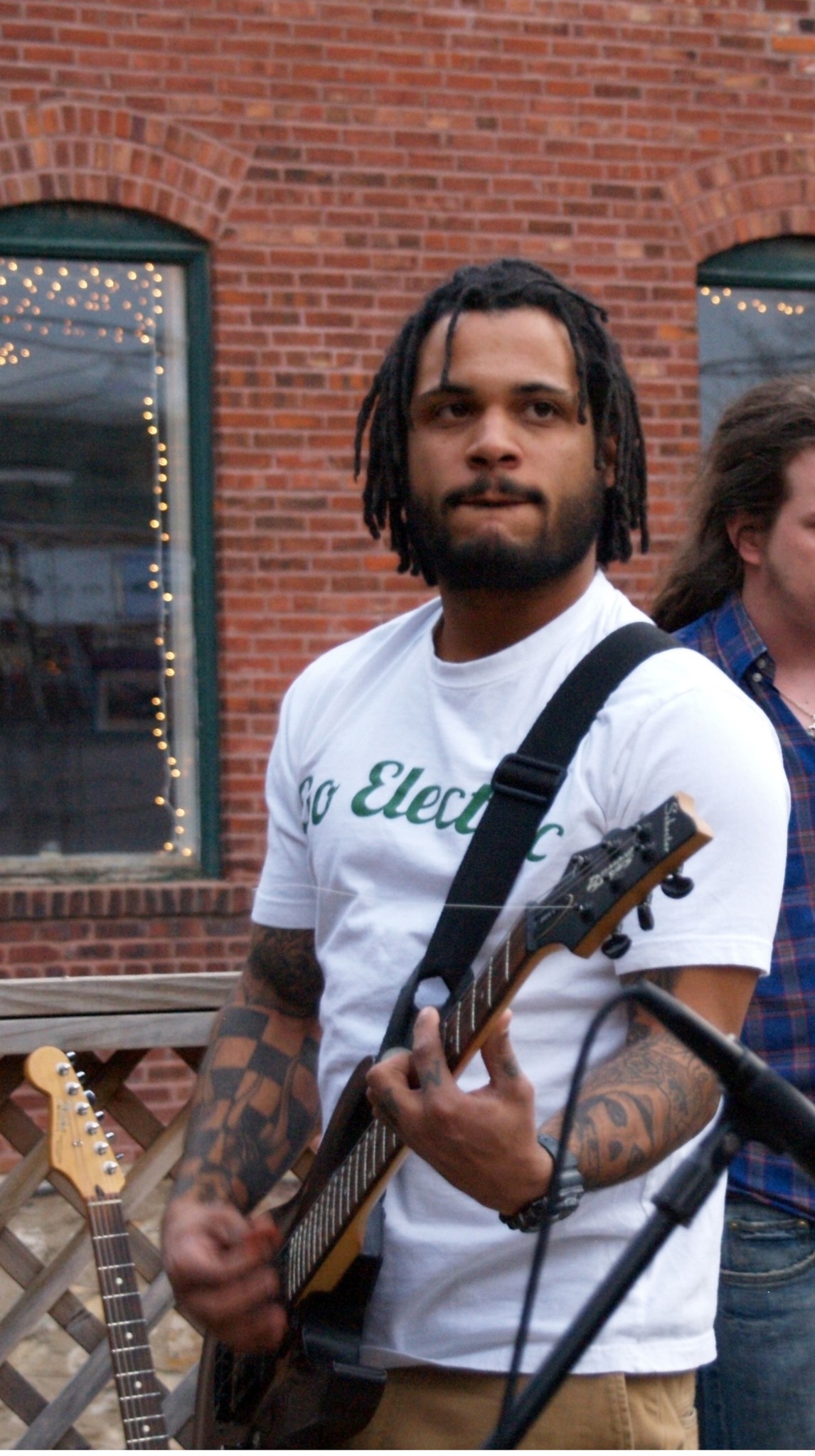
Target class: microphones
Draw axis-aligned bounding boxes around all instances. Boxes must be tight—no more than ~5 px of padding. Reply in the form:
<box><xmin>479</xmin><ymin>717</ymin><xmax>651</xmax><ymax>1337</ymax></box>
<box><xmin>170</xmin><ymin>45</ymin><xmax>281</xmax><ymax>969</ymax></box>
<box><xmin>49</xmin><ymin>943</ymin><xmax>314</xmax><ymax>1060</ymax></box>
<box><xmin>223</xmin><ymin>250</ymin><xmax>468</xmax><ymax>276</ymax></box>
<box><xmin>634</xmin><ymin>981</ymin><xmax>815</xmax><ymax>1182</ymax></box>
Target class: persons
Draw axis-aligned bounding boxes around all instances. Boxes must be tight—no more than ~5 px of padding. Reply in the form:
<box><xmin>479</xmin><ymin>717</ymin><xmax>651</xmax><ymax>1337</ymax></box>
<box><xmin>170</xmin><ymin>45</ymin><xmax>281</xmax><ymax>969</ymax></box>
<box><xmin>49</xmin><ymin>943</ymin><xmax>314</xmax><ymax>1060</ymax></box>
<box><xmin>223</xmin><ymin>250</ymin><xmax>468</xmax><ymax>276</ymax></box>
<box><xmin>158</xmin><ymin>253</ymin><xmax>795</xmax><ymax>1450</ymax></box>
<box><xmin>643</xmin><ymin>376</ymin><xmax>815</xmax><ymax>1450</ymax></box>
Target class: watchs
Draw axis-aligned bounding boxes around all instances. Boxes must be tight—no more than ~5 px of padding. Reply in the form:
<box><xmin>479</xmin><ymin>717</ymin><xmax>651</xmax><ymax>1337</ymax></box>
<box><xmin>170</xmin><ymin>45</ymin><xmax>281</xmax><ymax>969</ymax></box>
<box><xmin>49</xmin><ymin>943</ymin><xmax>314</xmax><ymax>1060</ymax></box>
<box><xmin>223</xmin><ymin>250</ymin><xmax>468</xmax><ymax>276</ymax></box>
<box><xmin>497</xmin><ymin>1131</ymin><xmax>582</xmax><ymax>1235</ymax></box>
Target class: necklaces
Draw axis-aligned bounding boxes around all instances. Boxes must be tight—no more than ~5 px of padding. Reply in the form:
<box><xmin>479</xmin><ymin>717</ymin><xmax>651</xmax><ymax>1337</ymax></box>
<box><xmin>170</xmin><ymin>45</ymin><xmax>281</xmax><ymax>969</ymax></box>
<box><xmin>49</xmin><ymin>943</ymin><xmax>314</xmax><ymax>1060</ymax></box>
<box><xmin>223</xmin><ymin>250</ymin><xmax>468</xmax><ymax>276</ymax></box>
<box><xmin>778</xmin><ymin>686</ymin><xmax>815</xmax><ymax>737</ymax></box>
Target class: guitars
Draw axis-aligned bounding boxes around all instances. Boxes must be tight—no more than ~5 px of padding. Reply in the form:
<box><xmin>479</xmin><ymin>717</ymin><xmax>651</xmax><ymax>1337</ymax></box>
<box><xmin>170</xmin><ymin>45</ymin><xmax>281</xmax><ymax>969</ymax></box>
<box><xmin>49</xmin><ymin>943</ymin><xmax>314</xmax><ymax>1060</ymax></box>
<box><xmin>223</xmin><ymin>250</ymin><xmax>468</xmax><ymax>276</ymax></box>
<box><xmin>192</xmin><ymin>790</ymin><xmax>713</xmax><ymax>1450</ymax></box>
<box><xmin>25</xmin><ymin>1045</ymin><xmax>170</xmax><ymax>1450</ymax></box>
